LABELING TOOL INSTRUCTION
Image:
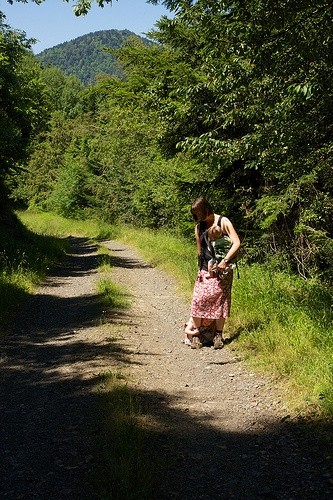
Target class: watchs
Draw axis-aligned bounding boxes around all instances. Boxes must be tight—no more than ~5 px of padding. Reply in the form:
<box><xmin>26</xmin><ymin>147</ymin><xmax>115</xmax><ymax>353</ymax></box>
<box><xmin>223</xmin><ymin>258</ymin><xmax>229</xmax><ymax>264</ymax></box>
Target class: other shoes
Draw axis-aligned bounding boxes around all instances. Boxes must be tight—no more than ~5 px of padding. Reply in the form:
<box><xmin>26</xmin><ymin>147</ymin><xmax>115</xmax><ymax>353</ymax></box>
<box><xmin>213</xmin><ymin>335</ymin><xmax>223</xmax><ymax>349</ymax></box>
<box><xmin>191</xmin><ymin>337</ymin><xmax>202</xmax><ymax>348</ymax></box>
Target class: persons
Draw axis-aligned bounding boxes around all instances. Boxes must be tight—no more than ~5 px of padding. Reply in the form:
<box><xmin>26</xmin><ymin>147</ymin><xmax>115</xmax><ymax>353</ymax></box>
<box><xmin>185</xmin><ymin>197</ymin><xmax>242</xmax><ymax>349</ymax></box>
<box><xmin>204</xmin><ymin>226</ymin><xmax>241</xmax><ymax>279</ymax></box>
<box><xmin>183</xmin><ymin>317</ymin><xmax>216</xmax><ymax>345</ymax></box>
